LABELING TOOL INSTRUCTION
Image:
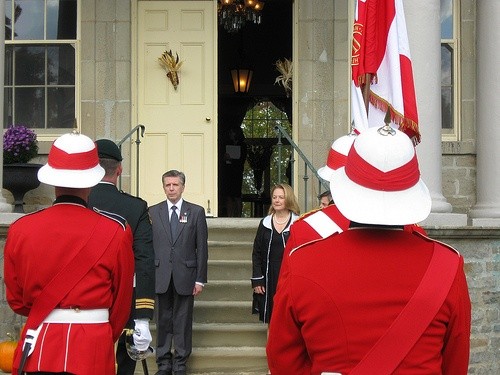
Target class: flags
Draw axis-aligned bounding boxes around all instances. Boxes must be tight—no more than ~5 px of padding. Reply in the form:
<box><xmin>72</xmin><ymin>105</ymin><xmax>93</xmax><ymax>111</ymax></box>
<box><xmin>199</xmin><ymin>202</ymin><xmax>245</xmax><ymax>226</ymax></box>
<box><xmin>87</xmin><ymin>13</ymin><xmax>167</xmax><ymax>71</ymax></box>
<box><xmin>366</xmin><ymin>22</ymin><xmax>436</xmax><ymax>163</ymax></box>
<box><xmin>350</xmin><ymin>0</ymin><xmax>422</xmax><ymax>148</ymax></box>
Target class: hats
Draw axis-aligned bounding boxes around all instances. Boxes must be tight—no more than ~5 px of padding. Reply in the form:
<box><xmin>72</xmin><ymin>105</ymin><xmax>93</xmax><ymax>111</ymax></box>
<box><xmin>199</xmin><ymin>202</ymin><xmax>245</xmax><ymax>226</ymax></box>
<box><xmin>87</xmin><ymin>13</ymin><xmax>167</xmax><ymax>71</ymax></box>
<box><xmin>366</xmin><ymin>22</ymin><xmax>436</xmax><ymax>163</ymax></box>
<box><xmin>37</xmin><ymin>131</ymin><xmax>107</xmax><ymax>189</ymax></box>
<box><xmin>330</xmin><ymin>104</ymin><xmax>431</xmax><ymax>226</ymax></box>
<box><xmin>317</xmin><ymin>134</ymin><xmax>358</xmax><ymax>182</ymax></box>
<box><xmin>92</xmin><ymin>139</ymin><xmax>122</xmax><ymax>161</ymax></box>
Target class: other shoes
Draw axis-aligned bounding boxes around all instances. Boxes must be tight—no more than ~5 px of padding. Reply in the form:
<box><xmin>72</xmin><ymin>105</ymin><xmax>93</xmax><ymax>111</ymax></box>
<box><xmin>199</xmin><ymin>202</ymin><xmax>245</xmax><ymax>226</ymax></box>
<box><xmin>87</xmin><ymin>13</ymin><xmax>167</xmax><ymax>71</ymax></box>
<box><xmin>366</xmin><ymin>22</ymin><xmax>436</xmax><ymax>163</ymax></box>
<box><xmin>154</xmin><ymin>370</ymin><xmax>172</xmax><ymax>375</ymax></box>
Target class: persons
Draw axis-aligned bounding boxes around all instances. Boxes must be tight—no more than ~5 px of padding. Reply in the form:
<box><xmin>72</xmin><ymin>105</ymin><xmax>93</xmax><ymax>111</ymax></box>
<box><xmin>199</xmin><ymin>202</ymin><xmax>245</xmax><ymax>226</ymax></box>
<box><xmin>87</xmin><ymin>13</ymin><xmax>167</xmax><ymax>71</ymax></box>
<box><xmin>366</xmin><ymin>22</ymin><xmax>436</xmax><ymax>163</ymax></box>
<box><xmin>88</xmin><ymin>139</ymin><xmax>155</xmax><ymax>375</ymax></box>
<box><xmin>3</xmin><ymin>119</ymin><xmax>136</xmax><ymax>375</ymax></box>
<box><xmin>251</xmin><ymin>184</ymin><xmax>302</xmax><ymax>341</ymax></box>
<box><xmin>317</xmin><ymin>191</ymin><xmax>332</xmax><ymax>210</ymax></box>
<box><xmin>265</xmin><ymin>106</ymin><xmax>473</xmax><ymax>375</ymax></box>
<box><xmin>148</xmin><ymin>170</ymin><xmax>208</xmax><ymax>375</ymax></box>
<box><xmin>272</xmin><ymin>116</ymin><xmax>360</xmax><ymax>316</ymax></box>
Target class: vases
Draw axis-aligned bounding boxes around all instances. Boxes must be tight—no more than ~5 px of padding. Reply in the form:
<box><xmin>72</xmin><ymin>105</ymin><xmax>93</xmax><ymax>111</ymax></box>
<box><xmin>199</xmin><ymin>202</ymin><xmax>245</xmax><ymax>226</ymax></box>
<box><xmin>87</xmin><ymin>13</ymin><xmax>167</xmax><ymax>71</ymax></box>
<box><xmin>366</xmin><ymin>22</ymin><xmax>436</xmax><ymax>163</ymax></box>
<box><xmin>3</xmin><ymin>164</ymin><xmax>46</xmax><ymax>202</ymax></box>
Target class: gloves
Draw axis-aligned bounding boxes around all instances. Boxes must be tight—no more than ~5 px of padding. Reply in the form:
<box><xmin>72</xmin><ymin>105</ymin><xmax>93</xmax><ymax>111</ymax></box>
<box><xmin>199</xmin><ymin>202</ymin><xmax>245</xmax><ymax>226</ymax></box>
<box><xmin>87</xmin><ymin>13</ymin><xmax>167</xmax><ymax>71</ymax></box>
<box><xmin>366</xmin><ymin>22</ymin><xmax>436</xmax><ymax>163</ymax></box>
<box><xmin>133</xmin><ymin>318</ymin><xmax>152</xmax><ymax>352</ymax></box>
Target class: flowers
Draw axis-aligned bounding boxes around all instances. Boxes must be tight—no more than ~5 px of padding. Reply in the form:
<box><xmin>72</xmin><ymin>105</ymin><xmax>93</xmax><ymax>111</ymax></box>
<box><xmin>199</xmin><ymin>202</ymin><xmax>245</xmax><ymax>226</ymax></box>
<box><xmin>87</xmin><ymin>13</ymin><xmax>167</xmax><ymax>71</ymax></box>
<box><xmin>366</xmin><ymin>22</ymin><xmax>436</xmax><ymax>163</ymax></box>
<box><xmin>4</xmin><ymin>126</ymin><xmax>42</xmax><ymax>165</ymax></box>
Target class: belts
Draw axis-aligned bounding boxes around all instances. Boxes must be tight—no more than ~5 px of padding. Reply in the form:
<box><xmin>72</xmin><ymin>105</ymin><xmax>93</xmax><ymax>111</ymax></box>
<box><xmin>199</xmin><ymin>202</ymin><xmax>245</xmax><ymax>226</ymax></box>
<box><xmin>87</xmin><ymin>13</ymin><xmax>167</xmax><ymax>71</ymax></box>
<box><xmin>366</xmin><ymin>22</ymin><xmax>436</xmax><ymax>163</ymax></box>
<box><xmin>45</xmin><ymin>308</ymin><xmax>111</xmax><ymax>325</ymax></box>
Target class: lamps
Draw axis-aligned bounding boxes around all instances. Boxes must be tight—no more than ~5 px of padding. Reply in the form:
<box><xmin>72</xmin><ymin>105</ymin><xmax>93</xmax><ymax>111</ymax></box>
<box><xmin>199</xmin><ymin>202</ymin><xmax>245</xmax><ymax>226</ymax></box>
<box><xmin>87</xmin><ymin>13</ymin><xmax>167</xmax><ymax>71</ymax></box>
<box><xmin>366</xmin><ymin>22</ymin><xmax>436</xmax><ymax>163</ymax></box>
<box><xmin>228</xmin><ymin>65</ymin><xmax>257</xmax><ymax>98</ymax></box>
<box><xmin>218</xmin><ymin>0</ymin><xmax>266</xmax><ymax>35</ymax></box>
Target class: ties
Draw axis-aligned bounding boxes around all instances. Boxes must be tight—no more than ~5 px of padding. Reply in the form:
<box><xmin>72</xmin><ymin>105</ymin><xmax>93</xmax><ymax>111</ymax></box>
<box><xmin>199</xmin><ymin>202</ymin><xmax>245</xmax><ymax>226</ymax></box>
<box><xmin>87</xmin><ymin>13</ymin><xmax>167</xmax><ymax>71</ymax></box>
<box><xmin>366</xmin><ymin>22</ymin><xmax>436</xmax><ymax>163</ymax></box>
<box><xmin>169</xmin><ymin>206</ymin><xmax>180</xmax><ymax>244</ymax></box>
